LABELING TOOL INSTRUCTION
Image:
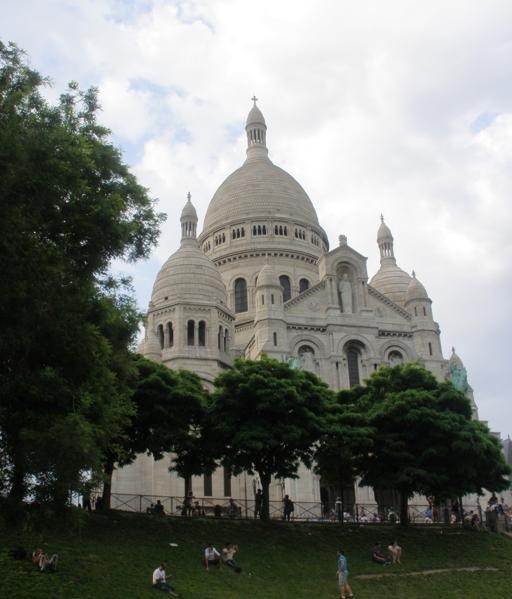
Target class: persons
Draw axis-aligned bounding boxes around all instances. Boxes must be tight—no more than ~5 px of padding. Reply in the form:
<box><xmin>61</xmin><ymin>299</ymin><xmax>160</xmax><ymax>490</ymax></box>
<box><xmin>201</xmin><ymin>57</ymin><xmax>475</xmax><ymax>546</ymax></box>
<box><xmin>254</xmin><ymin>489</ymin><xmax>262</xmax><ymax>520</ymax></box>
<box><xmin>372</xmin><ymin>541</ymin><xmax>385</xmax><ymax>563</ymax></box>
<box><xmin>32</xmin><ymin>547</ymin><xmax>59</xmax><ymax>572</ymax></box>
<box><xmin>425</xmin><ymin>491</ymin><xmax>511</xmax><ymax>533</ymax></box>
<box><xmin>202</xmin><ymin>543</ymin><xmax>241</xmax><ymax>573</ymax></box>
<box><xmin>152</xmin><ymin>562</ymin><xmax>181</xmax><ymax>597</ymax></box>
<box><xmin>338</xmin><ymin>271</ymin><xmax>353</xmax><ymax>311</ymax></box>
<box><xmin>83</xmin><ymin>481</ymin><xmax>92</xmax><ymax>511</ymax></box>
<box><xmin>335</xmin><ymin>497</ymin><xmax>343</xmax><ymax>519</ymax></box>
<box><xmin>146</xmin><ymin>491</ymin><xmax>221</xmax><ymax>518</ymax></box>
<box><xmin>387</xmin><ymin>540</ymin><xmax>402</xmax><ymax>564</ymax></box>
<box><xmin>335</xmin><ymin>548</ymin><xmax>353</xmax><ymax>599</ymax></box>
<box><xmin>282</xmin><ymin>495</ymin><xmax>292</xmax><ymax>520</ymax></box>
